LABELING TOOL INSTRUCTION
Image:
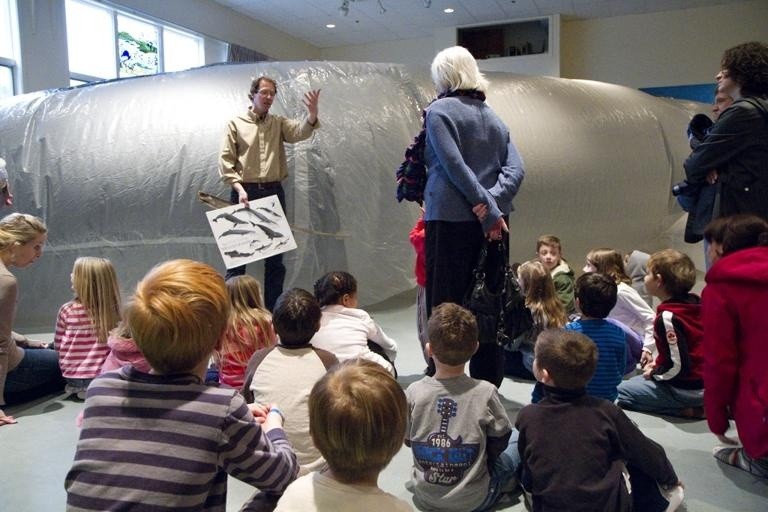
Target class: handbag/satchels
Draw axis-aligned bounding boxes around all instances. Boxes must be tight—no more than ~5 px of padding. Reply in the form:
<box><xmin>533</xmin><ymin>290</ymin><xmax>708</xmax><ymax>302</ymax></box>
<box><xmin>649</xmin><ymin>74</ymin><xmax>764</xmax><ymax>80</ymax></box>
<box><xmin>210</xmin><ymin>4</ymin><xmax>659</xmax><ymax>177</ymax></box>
<box><xmin>460</xmin><ymin>231</ymin><xmax>534</xmax><ymax>354</ymax></box>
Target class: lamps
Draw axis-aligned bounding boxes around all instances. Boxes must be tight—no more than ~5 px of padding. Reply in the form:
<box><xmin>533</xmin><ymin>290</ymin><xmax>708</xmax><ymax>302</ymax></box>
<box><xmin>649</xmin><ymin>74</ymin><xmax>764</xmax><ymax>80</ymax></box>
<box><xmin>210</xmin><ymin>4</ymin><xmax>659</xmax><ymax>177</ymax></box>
<box><xmin>336</xmin><ymin>0</ymin><xmax>433</xmax><ymax>18</ymax></box>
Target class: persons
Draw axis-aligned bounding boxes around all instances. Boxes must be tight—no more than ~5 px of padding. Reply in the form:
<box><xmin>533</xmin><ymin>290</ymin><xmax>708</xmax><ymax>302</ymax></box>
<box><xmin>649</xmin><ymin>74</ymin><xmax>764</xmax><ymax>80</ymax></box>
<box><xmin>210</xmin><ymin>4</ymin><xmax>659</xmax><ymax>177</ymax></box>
<box><xmin>682</xmin><ymin>42</ymin><xmax>768</xmax><ymax>223</ymax></box>
<box><xmin>218</xmin><ymin>77</ymin><xmax>321</xmax><ymax>313</ymax></box>
<box><xmin>703</xmin><ymin>89</ymin><xmax>733</xmax><ymax>271</ymax></box>
<box><xmin>396</xmin><ymin>45</ymin><xmax>525</xmax><ymax>387</ymax></box>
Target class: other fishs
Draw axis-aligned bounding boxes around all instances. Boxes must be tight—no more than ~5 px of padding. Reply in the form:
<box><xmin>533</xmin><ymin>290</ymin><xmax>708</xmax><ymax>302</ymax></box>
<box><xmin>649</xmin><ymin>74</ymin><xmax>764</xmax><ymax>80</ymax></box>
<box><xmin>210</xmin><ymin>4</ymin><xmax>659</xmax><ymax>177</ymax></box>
<box><xmin>213</xmin><ymin>202</ymin><xmax>289</xmax><ymax>258</ymax></box>
